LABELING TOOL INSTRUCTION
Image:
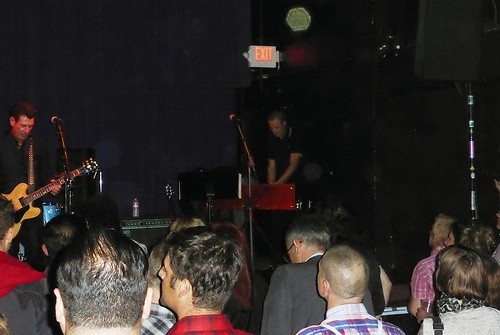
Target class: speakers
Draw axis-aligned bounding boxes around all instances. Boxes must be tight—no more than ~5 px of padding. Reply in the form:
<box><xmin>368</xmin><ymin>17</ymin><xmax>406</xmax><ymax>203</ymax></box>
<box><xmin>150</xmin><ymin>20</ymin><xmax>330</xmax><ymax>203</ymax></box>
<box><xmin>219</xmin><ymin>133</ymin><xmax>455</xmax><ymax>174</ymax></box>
<box><xmin>120</xmin><ymin>217</ymin><xmax>174</xmax><ymax>256</ymax></box>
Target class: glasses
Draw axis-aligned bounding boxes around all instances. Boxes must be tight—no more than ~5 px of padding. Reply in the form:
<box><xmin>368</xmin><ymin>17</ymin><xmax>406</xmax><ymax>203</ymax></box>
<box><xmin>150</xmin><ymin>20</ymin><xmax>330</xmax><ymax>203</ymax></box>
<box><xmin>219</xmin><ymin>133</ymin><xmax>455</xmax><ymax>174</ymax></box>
<box><xmin>283</xmin><ymin>241</ymin><xmax>303</xmax><ymax>263</ymax></box>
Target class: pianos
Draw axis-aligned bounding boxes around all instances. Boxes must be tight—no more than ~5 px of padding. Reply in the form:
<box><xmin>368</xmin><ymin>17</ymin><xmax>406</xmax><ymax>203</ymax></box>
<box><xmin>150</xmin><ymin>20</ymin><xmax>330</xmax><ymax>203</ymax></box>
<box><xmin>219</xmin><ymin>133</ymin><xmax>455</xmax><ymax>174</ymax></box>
<box><xmin>196</xmin><ymin>182</ymin><xmax>312</xmax><ymax>213</ymax></box>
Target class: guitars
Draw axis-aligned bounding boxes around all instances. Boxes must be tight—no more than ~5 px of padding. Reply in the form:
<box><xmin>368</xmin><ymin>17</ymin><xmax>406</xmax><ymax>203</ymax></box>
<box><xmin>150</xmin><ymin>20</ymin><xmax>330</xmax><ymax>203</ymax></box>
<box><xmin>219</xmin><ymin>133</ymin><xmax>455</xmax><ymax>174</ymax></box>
<box><xmin>0</xmin><ymin>156</ymin><xmax>98</xmax><ymax>242</ymax></box>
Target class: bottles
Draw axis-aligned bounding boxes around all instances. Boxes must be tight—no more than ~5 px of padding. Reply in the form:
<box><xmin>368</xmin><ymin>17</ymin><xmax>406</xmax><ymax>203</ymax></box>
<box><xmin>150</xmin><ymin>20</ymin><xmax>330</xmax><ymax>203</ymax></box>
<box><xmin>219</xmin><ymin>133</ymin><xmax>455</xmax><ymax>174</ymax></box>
<box><xmin>132</xmin><ymin>198</ymin><xmax>140</xmax><ymax>217</ymax></box>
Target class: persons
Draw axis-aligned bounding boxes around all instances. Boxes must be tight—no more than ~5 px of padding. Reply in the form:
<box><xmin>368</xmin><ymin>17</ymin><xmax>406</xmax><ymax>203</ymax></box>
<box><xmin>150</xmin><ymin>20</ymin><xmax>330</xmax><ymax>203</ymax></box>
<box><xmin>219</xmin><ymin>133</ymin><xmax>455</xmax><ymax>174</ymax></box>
<box><xmin>0</xmin><ymin>190</ymin><xmax>500</xmax><ymax>335</ymax></box>
<box><xmin>255</xmin><ymin>111</ymin><xmax>312</xmax><ymax>271</ymax></box>
<box><xmin>0</xmin><ymin>101</ymin><xmax>62</xmax><ymax>261</ymax></box>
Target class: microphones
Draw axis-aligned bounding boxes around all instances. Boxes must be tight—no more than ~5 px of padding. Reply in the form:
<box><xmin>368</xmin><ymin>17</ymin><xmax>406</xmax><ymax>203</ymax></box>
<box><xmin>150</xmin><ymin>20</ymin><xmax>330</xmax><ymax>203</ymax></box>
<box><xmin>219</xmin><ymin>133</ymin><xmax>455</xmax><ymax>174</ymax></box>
<box><xmin>230</xmin><ymin>114</ymin><xmax>240</xmax><ymax>122</ymax></box>
<box><xmin>51</xmin><ymin>117</ymin><xmax>62</xmax><ymax>124</ymax></box>
